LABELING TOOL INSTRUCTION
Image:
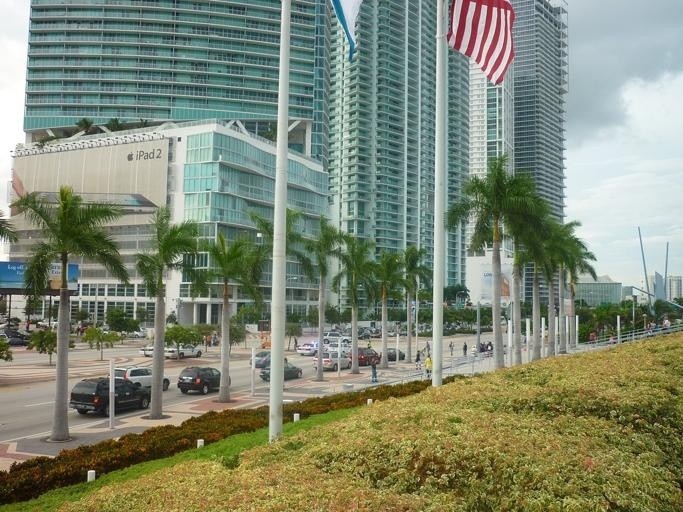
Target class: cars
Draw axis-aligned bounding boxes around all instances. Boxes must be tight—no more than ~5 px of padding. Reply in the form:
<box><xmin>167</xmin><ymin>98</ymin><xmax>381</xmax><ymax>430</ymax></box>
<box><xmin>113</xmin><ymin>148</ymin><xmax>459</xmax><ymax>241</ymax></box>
<box><xmin>138</xmin><ymin>342</ymin><xmax>202</xmax><ymax>360</ymax></box>
<box><xmin>296</xmin><ymin>331</ymin><xmax>405</xmax><ymax>372</ymax></box>
<box><xmin>121</xmin><ymin>326</ymin><xmax>148</xmax><ymax>338</ymax></box>
<box><xmin>37</xmin><ymin>318</ymin><xmax>57</xmax><ymax>330</ymax></box>
<box><xmin>249</xmin><ymin>351</ymin><xmax>303</xmax><ymax>382</ymax></box>
<box><xmin>0</xmin><ymin>316</ymin><xmax>33</xmax><ymax>347</ymax></box>
<box><xmin>356</xmin><ymin>322</ymin><xmax>470</xmax><ymax>340</ymax></box>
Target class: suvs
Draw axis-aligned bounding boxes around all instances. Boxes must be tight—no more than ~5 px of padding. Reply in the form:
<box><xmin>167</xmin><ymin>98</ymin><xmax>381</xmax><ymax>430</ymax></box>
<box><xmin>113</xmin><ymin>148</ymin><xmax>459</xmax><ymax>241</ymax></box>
<box><xmin>176</xmin><ymin>366</ymin><xmax>231</xmax><ymax>395</ymax></box>
<box><xmin>69</xmin><ymin>365</ymin><xmax>171</xmax><ymax>418</ymax></box>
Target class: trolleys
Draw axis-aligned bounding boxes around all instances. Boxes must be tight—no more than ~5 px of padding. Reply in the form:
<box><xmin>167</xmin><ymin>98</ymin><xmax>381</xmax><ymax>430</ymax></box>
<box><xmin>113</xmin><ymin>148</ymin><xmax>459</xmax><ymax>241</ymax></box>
<box><xmin>418</xmin><ymin>347</ymin><xmax>426</xmax><ymax>356</ymax></box>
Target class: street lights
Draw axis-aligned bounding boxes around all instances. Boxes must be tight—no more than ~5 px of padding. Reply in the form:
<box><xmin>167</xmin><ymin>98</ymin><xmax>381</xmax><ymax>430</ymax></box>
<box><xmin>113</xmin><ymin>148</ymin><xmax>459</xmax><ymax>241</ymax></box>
<box><xmin>455</xmin><ymin>291</ymin><xmax>465</xmax><ymax>310</ymax></box>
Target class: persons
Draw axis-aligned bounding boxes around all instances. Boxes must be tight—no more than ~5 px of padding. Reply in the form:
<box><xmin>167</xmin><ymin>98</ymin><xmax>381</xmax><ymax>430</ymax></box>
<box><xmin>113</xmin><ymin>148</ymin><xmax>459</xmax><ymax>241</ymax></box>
<box><xmin>371</xmin><ymin>359</ymin><xmax>378</xmax><ymax>382</ymax></box>
<box><xmin>415</xmin><ymin>341</ymin><xmax>432</xmax><ymax>378</ymax></box>
<box><xmin>463</xmin><ymin>341</ymin><xmax>467</xmax><ymax>355</ymax></box>
<box><xmin>25</xmin><ymin>321</ymin><xmax>29</xmax><ymax>330</ymax></box>
<box><xmin>294</xmin><ymin>337</ymin><xmax>301</xmax><ymax>351</ymax></box>
<box><xmin>646</xmin><ymin>315</ymin><xmax>672</xmax><ymax>329</ymax></box>
<box><xmin>589</xmin><ymin>330</ymin><xmax>597</xmax><ymax>344</ymax></box>
<box><xmin>449</xmin><ymin>341</ymin><xmax>454</xmax><ymax>356</ymax></box>
<box><xmin>202</xmin><ymin>333</ymin><xmax>218</xmax><ymax>347</ymax></box>
<box><xmin>368</xmin><ymin>340</ymin><xmax>371</xmax><ymax>349</ymax></box>
<box><xmin>480</xmin><ymin>341</ymin><xmax>493</xmax><ymax>352</ymax></box>
<box><xmin>75</xmin><ymin>321</ymin><xmax>87</xmax><ymax>336</ymax></box>
<box><xmin>608</xmin><ymin>332</ymin><xmax>617</xmax><ymax>344</ymax></box>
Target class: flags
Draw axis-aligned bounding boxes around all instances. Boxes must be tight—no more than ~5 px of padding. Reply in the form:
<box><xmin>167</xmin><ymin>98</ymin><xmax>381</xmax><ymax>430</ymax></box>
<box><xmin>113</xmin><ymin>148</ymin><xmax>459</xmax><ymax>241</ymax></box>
<box><xmin>330</xmin><ymin>0</ymin><xmax>364</xmax><ymax>63</ymax></box>
<box><xmin>445</xmin><ymin>0</ymin><xmax>516</xmax><ymax>86</ymax></box>
